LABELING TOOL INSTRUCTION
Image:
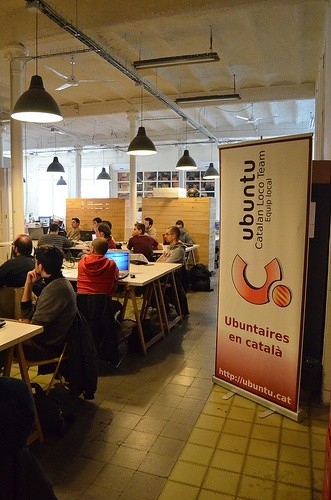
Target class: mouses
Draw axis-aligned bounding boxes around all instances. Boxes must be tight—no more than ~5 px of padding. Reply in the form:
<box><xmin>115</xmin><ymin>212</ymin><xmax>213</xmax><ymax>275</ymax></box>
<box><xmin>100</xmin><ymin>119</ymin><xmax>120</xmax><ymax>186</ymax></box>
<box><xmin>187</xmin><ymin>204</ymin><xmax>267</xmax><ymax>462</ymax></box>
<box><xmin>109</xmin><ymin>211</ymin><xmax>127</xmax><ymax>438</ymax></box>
<box><xmin>130</xmin><ymin>274</ymin><xmax>135</xmax><ymax>278</ymax></box>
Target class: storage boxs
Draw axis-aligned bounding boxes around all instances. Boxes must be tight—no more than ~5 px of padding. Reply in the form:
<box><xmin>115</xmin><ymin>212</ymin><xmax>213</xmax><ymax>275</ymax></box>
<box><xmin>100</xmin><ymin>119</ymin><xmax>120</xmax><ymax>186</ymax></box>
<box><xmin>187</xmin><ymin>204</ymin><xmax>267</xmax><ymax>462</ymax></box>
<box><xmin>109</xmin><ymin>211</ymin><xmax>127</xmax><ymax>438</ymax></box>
<box><xmin>153</xmin><ymin>187</ymin><xmax>187</xmax><ymax>198</ymax></box>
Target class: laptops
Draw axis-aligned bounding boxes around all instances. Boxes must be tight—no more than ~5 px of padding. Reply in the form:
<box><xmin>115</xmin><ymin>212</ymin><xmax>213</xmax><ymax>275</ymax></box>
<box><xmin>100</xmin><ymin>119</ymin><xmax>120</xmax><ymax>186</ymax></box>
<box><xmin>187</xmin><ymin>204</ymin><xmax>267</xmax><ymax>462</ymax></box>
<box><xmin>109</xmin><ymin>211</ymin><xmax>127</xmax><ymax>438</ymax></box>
<box><xmin>104</xmin><ymin>249</ymin><xmax>130</xmax><ymax>278</ymax></box>
<box><xmin>28</xmin><ymin>227</ymin><xmax>44</xmax><ymax>240</ymax></box>
<box><xmin>80</xmin><ymin>230</ymin><xmax>95</xmax><ymax>242</ymax></box>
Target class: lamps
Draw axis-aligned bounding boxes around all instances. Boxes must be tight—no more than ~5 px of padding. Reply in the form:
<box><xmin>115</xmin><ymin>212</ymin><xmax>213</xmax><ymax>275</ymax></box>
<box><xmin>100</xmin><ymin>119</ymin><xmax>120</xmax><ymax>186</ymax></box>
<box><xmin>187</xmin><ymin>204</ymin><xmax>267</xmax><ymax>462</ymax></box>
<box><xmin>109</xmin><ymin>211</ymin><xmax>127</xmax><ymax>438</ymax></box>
<box><xmin>176</xmin><ymin>73</ymin><xmax>241</xmax><ymax>104</ymax></box>
<box><xmin>11</xmin><ymin>0</ymin><xmax>64</xmax><ymax>123</ymax></box>
<box><xmin>97</xmin><ymin>150</ymin><xmax>112</xmax><ymax>181</ymax></box>
<box><xmin>203</xmin><ymin>140</ymin><xmax>220</xmax><ymax>179</ymax></box>
<box><xmin>133</xmin><ymin>26</ymin><xmax>220</xmax><ymax>69</ymax></box>
<box><xmin>46</xmin><ymin>128</ymin><xmax>66</xmax><ymax>173</ymax></box>
<box><xmin>127</xmin><ymin>82</ymin><xmax>157</xmax><ymax>155</ymax></box>
<box><xmin>57</xmin><ymin>176</ymin><xmax>67</xmax><ymax>185</ymax></box>
<box><xmin>175</xmin><ymin>117</ymin><xmax>198</xmax><ymax>170</ymax></box>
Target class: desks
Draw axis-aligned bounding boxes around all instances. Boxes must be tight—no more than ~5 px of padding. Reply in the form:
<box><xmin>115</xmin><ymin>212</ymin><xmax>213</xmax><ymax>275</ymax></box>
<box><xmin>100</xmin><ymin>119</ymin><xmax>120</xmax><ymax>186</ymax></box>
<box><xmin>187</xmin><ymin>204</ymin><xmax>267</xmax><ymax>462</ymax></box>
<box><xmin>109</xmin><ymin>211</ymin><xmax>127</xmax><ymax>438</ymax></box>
<box><xmin>64</xmin><ymin>241</ymin><xmax>127</xmax><ymax>250</ymax></box>
<box><xmin>121</xmin><ymin>243</ymin><xmax>199</xmax><ymax>266</ymax></box>
<box><xmin>0</xmin><ymin>321</ymin><xmax>44</xmax><ymax>445</ymax></box>
<box><xmin>61</xmin><ymin>262</ymin><xmax>183</xmax><ymax>359</ymax></box>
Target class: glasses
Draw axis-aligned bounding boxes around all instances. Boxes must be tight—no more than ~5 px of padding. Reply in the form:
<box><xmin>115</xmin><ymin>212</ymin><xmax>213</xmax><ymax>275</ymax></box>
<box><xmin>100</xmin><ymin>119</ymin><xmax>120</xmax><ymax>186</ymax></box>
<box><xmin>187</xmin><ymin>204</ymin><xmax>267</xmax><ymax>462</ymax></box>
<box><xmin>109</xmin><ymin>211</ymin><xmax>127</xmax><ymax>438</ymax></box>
<box><xmin>166</xmin><ymin>233</ymin><xmax>173</xmax><ymax>236</ymax></box>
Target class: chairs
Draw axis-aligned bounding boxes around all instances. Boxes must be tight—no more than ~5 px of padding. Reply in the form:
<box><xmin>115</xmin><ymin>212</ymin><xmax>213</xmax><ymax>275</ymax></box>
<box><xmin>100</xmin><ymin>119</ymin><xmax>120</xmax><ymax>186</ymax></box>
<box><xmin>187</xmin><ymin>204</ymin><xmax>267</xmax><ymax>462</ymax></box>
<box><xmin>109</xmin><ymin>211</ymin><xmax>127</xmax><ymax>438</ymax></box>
<box><xmin>12</xmin><ymin>310</ymin><xmax>99</xmax><ymax>399</ymax></box>
<box><xmin>0</xmin><ymin>287</ymin><xmax>22</xmax><ymax>320</ymax></box>
<box><xmin>129</xmin><ymin>253</ymin><xmax>149</xmax><ymax>262</ymax></box>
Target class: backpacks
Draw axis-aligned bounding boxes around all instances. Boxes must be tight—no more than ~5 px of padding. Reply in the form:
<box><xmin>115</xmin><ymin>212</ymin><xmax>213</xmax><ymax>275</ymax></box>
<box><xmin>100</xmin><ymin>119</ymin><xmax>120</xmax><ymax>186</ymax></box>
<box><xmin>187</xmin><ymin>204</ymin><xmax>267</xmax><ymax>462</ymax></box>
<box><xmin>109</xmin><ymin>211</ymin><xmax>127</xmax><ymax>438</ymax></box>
<box><xmin>189</xmin><ymin>264</ymin><xmax>213</xmax><ymax>292</ymax></box>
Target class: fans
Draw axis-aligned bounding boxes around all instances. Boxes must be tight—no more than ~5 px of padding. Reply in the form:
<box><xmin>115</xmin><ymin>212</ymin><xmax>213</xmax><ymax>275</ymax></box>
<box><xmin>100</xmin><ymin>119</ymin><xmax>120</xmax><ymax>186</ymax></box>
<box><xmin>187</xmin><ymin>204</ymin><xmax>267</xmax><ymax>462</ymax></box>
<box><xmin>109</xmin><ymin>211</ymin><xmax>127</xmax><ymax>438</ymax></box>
<box><xmin>235</xmin><ymin>103</ymin><xmax>280</xmax><ymax>123</ymax></box>
<box><xmin>45</xmin><ymin>52</ymin><xmax>114</xmax><ymax>91</ymax></box>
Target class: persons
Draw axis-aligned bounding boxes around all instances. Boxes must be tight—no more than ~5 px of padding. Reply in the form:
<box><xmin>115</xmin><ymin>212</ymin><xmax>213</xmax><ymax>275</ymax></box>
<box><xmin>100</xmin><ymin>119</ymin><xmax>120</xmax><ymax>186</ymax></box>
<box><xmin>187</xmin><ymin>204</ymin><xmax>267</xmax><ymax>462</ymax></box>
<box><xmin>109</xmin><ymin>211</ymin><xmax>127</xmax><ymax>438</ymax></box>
<box><xmin>0</xmin><ymin>234</ymin><xmax>42</xmax><ymax>312</ymax></box>
<box><xmin>51</xmin><ymin>219</ymin><xmax>66</xmax><ymax>238</ymax></box>
<box><xmin>147</xmin><ymin>226</ymin><xmax>186</xmax><ymax>301</ymax></box>
<box><xmin>36</xmin><ymin>223</ymin><xmax>75</xmax><ymax>262</ymax></box>
<box><xmin>170</xmin><ymin>222</ymin><xmax>192</xmax><ymax>246</ymax></box>
<box><xmin>65</xmin><ymin>218</ymin><xmax>81</xmax><ymax>258</ymax></box>
<box><xmin>81</xmin><ymin>223</ymin><xmax>116</xmax><ymax>258</ymax></box>
<box><xmin>90</xmin><ymin>217</ymin><xmax>113</xmax><ymax>244</ymax></box>
<box><xmin>0</xmin><ymin>243</ymin><xmax>78</xmax><ymax>370</ymax></box>
<box><xmin>127</xmin><ymin>223</ymin><xmax>163</xmax><ymax>262</ymax></box>
<box><xmin>75</xmin><ymin>237</ymin><xmax>136</xmax><ymax>374</ymax></box>
<box><xmin>143</xmin><ymin>217</ymin><xmax>157</xmax><ymax>239</ymax></box>
